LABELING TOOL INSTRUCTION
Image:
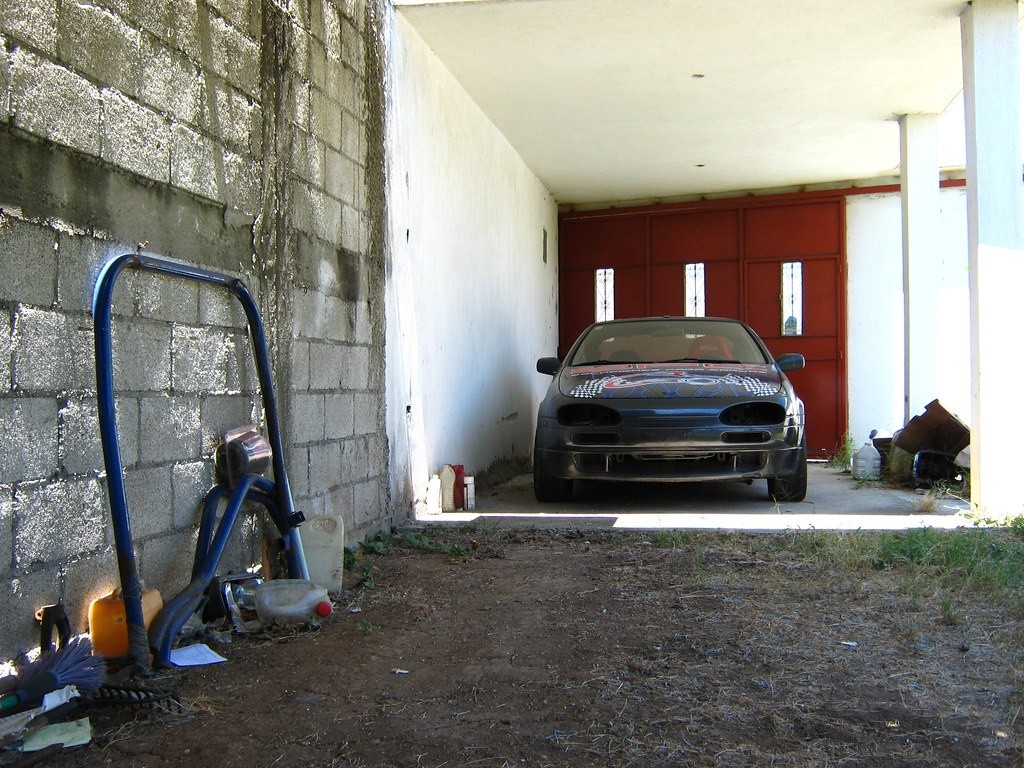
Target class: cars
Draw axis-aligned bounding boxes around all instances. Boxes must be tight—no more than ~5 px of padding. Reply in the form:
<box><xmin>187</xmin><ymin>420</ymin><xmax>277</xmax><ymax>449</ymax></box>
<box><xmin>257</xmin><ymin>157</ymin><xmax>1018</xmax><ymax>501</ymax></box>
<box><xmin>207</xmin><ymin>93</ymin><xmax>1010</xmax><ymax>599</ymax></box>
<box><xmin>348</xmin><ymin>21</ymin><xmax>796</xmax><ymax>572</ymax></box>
<box><xmin>532</xmin><ymin>315</ymin><xmax>808</xmax><ymax>504</ymax></box>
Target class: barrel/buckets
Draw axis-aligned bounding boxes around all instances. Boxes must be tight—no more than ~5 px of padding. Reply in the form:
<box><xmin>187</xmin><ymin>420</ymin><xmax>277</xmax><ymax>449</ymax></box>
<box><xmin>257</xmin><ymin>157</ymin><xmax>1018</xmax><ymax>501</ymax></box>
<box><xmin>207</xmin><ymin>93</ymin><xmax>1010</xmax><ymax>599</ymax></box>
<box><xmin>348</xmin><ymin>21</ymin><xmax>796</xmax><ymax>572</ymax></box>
<box><xmin>298</xmin><ymin>514</ymin><xmax>344</xmax><ymax>595</ymax></box>
<box><xmin>253</xmin><ymin>577</ymin><xmax>334</xmax><ymax>628</ymax></box>
<box><xmin>88</xmin><ymin>586</ymin><xmax>164</xmax><ymax>658</ymax></box>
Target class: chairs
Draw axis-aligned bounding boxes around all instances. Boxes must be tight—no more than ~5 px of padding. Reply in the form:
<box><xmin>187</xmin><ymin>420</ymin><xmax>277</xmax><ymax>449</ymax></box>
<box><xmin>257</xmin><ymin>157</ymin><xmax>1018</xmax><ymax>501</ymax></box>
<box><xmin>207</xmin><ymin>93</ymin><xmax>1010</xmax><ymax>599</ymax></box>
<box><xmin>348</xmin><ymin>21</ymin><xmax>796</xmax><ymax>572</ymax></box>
<box><xmin>611</xmin><ymin>350</ymin><xmax>640</xmax><ymax>360</ymax></box>
<box><xmin>689</xmin><ymin>335</ymin><xmax>731</xmax><ymax>359</ymax></box>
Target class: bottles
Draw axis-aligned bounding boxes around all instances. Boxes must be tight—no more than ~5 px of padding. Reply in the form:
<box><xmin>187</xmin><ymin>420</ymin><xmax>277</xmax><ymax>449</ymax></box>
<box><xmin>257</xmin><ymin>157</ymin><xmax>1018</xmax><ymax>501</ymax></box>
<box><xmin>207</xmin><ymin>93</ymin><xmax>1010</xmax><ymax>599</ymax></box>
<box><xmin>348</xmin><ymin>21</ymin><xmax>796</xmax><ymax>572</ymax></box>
<box><xmin>426</xmin><ymin>474</ymin><xmax>443</xmax><ymax>515</ymax></box>
<box><xmin>453</xmin><ymin>465</ymin><xmax>469</xmax><ymax>508</ymax></box>
<box><xmin>853</xmin><ymin>441</ymin><xmax>881</xmax><ymax>480</ymax></box>
<box><xmin>463</xmin><ymin>475</ymin><xmax>475</xmax><ymax>511</ymax></box>
<box><xmin>440</xmin><ymin>464</ymin><xmax>456</xmax><ymax>512</ymax></box>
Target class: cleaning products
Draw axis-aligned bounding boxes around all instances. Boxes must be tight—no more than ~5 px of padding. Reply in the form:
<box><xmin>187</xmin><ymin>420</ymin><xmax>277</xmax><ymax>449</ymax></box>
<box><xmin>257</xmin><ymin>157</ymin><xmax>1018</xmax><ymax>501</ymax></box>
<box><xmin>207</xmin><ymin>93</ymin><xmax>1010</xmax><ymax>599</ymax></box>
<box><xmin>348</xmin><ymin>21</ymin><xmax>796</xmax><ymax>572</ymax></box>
<box><xmin>429</xmin><ymin>465</ymin><xmax>475</xmax><ymax>514</ymax></box>
<box><xmin>852</xmin><ymin>443</ymin><xmax>881</xmax><ymax>480</ymax></box>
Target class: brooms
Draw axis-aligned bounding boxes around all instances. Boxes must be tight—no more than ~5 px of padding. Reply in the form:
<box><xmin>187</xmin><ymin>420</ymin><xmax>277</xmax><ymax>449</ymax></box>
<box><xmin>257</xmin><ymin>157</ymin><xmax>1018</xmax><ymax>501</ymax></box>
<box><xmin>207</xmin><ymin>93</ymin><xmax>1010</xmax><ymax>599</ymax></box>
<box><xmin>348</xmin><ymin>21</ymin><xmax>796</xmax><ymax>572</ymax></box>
<box><xmin>0</xmin><ymin>636</ymin><xmax>107</xmax><ymax>710</ymax></box>
